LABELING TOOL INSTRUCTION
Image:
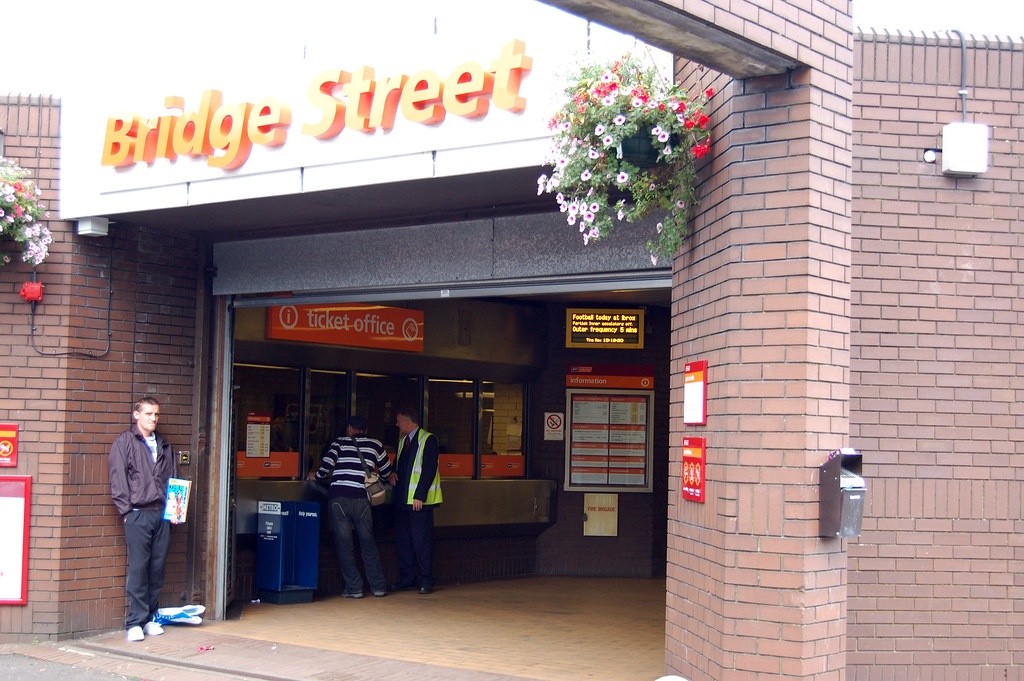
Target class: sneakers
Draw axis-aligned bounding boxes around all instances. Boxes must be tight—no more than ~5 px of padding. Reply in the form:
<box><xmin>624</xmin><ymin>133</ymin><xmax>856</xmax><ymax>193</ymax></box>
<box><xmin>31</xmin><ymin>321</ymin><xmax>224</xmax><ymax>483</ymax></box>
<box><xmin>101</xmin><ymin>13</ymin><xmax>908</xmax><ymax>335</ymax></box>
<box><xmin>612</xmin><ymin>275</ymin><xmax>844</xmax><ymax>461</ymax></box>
<box><xmin>143</xmin><ymin>622</ymin><xmax>164</xmax><ymax>635</ymax></box>
<box><xmin>128</xmin><ymin>626</ymin><xmax>145</xmax><ymax>641</ymax></box>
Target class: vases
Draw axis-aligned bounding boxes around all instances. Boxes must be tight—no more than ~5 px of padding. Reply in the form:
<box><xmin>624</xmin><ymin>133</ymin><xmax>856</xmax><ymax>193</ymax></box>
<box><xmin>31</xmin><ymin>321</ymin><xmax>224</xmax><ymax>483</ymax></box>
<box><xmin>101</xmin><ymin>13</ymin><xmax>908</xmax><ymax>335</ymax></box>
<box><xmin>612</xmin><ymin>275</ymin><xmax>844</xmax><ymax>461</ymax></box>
<box><xmin>612</xmin><ymin>121</ymin><xmax>684</xmax><ymax>169</ymax></box>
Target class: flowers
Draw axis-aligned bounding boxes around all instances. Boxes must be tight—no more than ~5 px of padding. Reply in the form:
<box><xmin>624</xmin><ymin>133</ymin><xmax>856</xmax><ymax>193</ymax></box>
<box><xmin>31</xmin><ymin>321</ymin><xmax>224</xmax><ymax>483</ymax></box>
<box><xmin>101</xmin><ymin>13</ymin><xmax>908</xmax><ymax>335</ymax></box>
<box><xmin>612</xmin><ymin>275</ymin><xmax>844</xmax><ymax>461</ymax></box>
<box><xmin>535</xmin><ymin>51</ymin><xmax>714</xmax><ymax>266</ymax></box>
<box><xmin>0</xmin><ymin>156</ymin><xmax>54</xmax><ymax>270</ymax></box>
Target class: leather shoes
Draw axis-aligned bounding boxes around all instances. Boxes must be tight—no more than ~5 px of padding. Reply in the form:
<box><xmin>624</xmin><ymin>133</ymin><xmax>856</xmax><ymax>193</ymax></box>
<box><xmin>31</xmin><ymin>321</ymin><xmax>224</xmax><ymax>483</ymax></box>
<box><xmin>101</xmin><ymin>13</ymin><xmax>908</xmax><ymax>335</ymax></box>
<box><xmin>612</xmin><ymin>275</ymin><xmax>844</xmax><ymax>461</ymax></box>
<box><xmin>419</xmin><ymin>584</ymin><xmax>431</xmax><ymax>594</ymax></box>
<box><xmin>390</xmin><ymin>583</ymin><xmax>411</xmax><ymax>591</ymax></box>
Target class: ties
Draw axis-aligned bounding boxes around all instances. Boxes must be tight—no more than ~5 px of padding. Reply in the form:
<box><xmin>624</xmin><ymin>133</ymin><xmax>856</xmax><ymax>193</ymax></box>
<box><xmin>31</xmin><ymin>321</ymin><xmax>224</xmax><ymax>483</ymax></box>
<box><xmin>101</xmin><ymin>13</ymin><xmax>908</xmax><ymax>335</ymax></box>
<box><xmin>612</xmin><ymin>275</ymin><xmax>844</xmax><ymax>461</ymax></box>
<box><xmin>405</xmin><ymin>435</ymin><xmax>410</xmax><ymax>449</ymax></box>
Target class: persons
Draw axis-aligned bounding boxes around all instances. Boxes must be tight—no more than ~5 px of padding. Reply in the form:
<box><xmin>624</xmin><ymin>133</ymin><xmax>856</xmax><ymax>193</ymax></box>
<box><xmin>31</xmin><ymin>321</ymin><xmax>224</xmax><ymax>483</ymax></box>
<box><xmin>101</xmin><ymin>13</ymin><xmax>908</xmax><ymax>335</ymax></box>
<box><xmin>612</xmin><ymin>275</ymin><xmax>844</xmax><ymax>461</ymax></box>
<box><xmin>307</xmin><ymin>417</ymin><xmax>391</xmax><ymax>599</ymax></box>
<box><xmin>391</xmin><ymin>407</ymin><xmax>444</xmax><ymax>594</ymax></box>
<box><xmin>109</xmin><ymin>398</ymin><xmax>179</xmax><ymax>641</ymax></box>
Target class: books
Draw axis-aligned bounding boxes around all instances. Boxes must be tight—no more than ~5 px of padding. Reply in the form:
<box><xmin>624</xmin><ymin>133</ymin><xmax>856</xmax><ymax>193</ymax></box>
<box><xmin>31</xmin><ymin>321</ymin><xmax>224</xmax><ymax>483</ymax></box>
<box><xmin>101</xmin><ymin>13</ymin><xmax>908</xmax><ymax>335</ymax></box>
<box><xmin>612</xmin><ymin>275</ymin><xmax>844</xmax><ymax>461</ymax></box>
<box><xmin>163</xmin><ymin>478</ymin><xmax>192</xmax><ymax>522</ymax></box>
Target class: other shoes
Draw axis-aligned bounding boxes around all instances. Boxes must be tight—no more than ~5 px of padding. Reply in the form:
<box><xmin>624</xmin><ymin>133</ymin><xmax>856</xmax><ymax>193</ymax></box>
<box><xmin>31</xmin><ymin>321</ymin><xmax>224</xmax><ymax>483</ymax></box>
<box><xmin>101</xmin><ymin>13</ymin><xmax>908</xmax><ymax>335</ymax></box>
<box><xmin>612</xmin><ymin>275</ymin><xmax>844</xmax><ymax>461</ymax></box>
<box><xmin>371</xmin><ymin>588</ymin><xmax>385</xmax><ymax>597</ymax></box>
<box><xmin>342</xmin><ymin>591</ymin><xmax>363</xmax><ymax>598</ymax></box>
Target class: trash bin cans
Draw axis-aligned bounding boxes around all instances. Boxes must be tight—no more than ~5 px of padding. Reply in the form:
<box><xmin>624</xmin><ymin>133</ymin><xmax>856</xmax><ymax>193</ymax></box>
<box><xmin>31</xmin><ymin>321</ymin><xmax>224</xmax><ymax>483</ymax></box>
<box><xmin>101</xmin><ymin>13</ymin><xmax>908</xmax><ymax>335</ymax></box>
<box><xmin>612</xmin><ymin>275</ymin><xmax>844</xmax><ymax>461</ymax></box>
<box><xmin>253</xmin><ymin>500</ymin><xmax>321</xmax><ymax>604</ymax></box>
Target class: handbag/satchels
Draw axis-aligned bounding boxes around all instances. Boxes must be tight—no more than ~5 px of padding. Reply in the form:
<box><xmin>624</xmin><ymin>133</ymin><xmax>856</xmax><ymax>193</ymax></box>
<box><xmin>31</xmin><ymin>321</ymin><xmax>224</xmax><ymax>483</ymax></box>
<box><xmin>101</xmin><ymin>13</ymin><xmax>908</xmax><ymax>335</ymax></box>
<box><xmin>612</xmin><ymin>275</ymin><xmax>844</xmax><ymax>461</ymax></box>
<box><xmin>365</xmin><ymin>472</ymin><xmax>392</xmax><ymax>506</ymax></box>
